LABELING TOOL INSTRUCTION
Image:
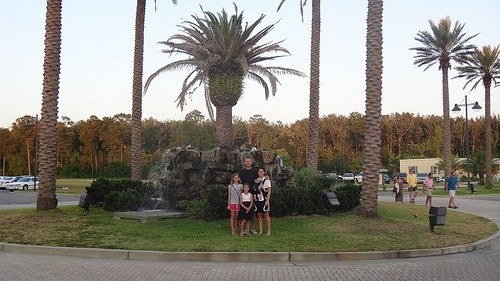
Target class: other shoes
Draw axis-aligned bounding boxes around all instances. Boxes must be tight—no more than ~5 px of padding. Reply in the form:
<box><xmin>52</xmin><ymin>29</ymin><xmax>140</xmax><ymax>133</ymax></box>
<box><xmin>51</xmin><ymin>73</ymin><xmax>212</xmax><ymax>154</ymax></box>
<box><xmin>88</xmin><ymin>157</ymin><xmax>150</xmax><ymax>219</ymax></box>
<box><xmin>246</xmin><ymin>232</ymin><xmax>251</xmax><ymax>237</ymax></box>
<box><xmin>265</xmin><ymin>232</ymin><xmax>272</xmax><ymax>236</ymax></box>
<box><xmin>449</xmin><ymin>205</ymin><xmax>458</xmax><ymax>208</ymax></box>
<box><xmin>257</xmin><ymin>232</ymin><xmax>263</xmax><ymax>236</ymax></box>
<box><xmin>251</xmin><ymin>228</ymin><xmax>258</xmax><ymax>235</ymax></box>
<box><xmin>239</xmin><ymin>232</ymin><xmax>244</xmax><ymax>237</ymax></box>
<box><xmin>410</xmin><ymin>199</ymin><xmax>415</xmax><ymax>203</ymax></box>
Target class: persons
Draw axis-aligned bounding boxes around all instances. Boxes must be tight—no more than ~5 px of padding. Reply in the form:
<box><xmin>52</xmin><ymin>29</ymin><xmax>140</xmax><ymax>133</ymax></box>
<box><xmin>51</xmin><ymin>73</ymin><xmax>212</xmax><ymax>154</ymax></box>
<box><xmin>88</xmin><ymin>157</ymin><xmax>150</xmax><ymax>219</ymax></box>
<box><xmin>239</xmin><ymin>183</ymin><xmax>253</xmax><ymax>237</ymax></box>
<box><xmin>226</xmin><ymin>173</ymin><xmax>244</xmax><ymax>236</ymax></box>
<box><xmin>252</xmin><ymin>168</ymin><xmax>271</xmax><ymax>236</ymax></box>
<box><xmin>231</xmin><ymin>157</ymin><xmax>270</xmax><ymax>235</ymax></box>
<box><xmin>422</xmin><ymin>172</ymin><xmax>435</xmax><ymax>207</ymax></box>
<box><xmin>406</xmin><ymin>168</ymin><xmax>418</xmax><ymax>202</ymax></box>
<box><xmin>446</xmin><ymin>171</ymin><xmax>462</xmax><ymax>209</ymax></box>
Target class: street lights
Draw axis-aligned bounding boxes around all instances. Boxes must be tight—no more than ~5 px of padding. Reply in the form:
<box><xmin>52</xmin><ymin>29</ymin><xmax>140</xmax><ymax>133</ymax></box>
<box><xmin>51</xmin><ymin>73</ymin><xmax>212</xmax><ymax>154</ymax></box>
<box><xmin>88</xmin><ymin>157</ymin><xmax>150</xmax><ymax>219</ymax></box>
<box><xmin>451</xmin><ymin>95</ymin><xmax>483</xmax><ymax>190</ymax></box>
<box><xmin>24</xmin><ymin>114</ymin><xmax>40</xmax><ymax>192</ymax></box>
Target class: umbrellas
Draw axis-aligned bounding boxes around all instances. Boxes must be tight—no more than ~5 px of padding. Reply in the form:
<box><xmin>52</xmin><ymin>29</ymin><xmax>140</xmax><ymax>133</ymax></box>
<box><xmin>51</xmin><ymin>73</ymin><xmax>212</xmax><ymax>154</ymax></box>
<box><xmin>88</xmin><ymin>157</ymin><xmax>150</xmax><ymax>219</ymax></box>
<box><xmin>394</xmin><ymin>173</ymin><xmax>407</xmax><ymax>179</ymax></box>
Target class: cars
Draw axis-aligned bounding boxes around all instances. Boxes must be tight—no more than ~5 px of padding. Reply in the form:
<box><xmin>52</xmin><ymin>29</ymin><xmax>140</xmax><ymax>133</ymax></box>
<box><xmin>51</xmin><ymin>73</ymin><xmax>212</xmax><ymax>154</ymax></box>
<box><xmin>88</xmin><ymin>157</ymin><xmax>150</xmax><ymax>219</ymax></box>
<box><xmin>384</xmin><ymin>175</ymin><xmax>390</xmax><ymax>184</ymax></box>
<box><xmin>317</xmin><ymin>171</ymin><xmax>363</xmax><ymax>184</ymax></box>
<box><xmin>394</xmin><ymin>172</ymin><xmax>408</xmax><ymax>184</ymax></box>
<box><xmin>6</xmin><ymin>177</ymin><xmax>39</xmax><ymax>192</ymax></box>
<box><xmin>0</xmin><ymin>175</ymin><xmax>23</xmax><ymax>190</ymax></box>
<box><xmin>416</xmin><ymin>172</ymin><xmax>428</xmax><ymax>184</ymax></box>
